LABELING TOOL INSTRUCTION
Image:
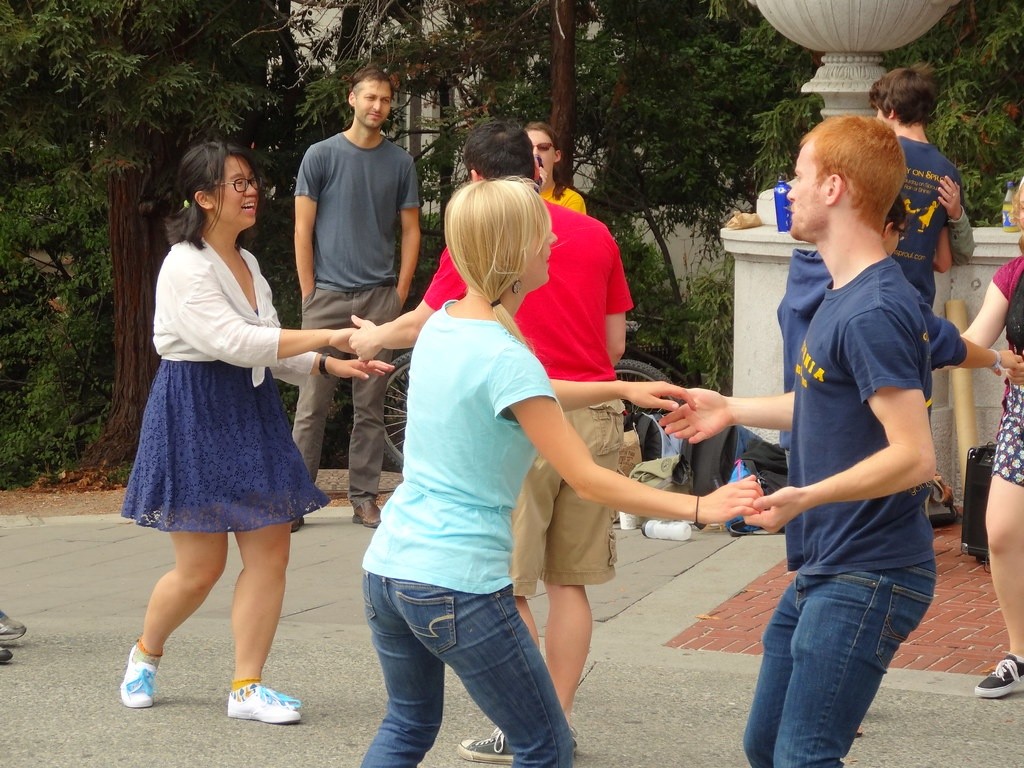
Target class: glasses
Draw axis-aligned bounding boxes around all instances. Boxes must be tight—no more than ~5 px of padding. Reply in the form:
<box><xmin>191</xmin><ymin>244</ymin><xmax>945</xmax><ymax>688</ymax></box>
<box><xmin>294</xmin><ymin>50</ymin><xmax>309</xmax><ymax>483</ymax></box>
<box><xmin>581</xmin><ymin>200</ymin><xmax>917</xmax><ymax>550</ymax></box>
<box><xmin>893</xmin><ymin>226</ymin><xmax>908</xmax><ymax>241</ymax></box>
<box><xmin>215</xmin><ymin>177</ymin><xmax>261</xmax><ymax>192</ymax></box>
<box><xmin>532</xmin><ymin>143</ymin><xmax>556</xmax><ymax>151</ymax></box>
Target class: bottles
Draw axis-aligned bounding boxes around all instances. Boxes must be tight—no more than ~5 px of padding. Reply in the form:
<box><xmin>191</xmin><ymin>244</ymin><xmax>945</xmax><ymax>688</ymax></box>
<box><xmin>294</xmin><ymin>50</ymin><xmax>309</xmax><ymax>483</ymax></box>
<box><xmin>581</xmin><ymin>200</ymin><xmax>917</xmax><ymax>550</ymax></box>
<box><xmin>641</xmin><ymin>518</ymin><xmax>691</xmax><ymax>541</ymax></box>
<box><xmin>709</xmin><ymin>476</ymin><xmax>724</xmax><ymax>492</ymax></box>
<box><xmin>1002</xmin><ymin>181</ymin><xmax>1022</xmax><ymax>232</ymax></box>
<box><xmin>775</xmin><ymin>172</ymin><xmax>792</xmax><ymax>232</ymax></box>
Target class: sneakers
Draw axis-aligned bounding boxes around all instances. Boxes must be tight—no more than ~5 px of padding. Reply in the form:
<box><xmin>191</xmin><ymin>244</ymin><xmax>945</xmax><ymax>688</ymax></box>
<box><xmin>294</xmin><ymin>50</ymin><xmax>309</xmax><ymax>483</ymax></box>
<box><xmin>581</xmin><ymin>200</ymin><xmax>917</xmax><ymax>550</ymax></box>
<box><xmin>458</xmin><ymin>727</ymin><xmax>577</xmax><ymax>764</ymax></box>
<box><xmin>120</xmin><ymin>645</ymin><xmax>157</xmax><ymax>707</ymax></box>
<box><xmin>0</xmin><ymin>610</ymin><xmax>26</xmax><ymax>662</ymax></box>
<box><xmin>227</xmin><ymin>683</ymin><xmax>302</xmax><ymax>724</ymax></box>
<box><xmin>975</xmin><ymin>654</ymin><xmax>1024</xmax><ymax>697</ymax></box>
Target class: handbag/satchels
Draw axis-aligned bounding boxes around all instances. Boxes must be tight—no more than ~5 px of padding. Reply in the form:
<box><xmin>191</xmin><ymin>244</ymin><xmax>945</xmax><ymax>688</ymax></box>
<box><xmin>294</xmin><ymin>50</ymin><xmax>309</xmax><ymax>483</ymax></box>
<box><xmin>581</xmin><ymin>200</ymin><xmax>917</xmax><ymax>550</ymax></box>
<box><xmin>924</xmin><ymin>473</ymin><xmax>958</xmax><ymax>528</ymax></box>
<box><xmin>627</xmin><ymin>454</ymin><xmax>693</xmax><ymax>529</ymax></box>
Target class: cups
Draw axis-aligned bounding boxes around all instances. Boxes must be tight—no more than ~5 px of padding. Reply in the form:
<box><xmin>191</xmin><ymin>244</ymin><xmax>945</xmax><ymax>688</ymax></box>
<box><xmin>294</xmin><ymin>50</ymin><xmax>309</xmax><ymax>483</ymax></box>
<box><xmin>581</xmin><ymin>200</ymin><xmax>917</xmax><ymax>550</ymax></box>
<box><xmin>618</xmin><ymin>510</ymin><xmax>637</xmax><ymax>530</ymax></box>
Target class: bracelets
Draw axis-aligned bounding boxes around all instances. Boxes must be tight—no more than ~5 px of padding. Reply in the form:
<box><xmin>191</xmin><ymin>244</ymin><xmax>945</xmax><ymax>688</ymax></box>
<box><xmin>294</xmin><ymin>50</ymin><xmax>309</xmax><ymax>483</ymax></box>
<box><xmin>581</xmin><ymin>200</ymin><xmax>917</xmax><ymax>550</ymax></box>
<box><xmin>693</xmin><ymin>495</ymin><xmax>702</xmax><ymax>523</ymax></box>
<box><xmin>989</xmin><ymin>348</ymin><xmax>1002</xmax><ymax>371</ymax></box>
<box><xmin>319</xmin><ymin>352</ymin><xmax>333</xmax><ymax>375</ymax></box>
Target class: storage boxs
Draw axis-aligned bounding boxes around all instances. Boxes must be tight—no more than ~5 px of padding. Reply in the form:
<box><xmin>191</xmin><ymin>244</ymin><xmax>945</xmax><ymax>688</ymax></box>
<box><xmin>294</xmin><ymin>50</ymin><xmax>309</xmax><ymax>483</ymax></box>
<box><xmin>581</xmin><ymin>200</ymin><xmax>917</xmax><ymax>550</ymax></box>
<box><xmin>616</xmin><ymin>421</ymin><xmax>643</xmax><ymax>479</ymax></box>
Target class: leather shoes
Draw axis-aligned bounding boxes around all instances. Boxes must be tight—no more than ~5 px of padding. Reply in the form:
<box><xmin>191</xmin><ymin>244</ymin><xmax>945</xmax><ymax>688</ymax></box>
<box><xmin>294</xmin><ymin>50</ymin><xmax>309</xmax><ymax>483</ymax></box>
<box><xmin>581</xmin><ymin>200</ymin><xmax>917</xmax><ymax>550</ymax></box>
<box><xmin>290</xmin><ymin>516</ymin><xmax>304</xmax><ymax>532</ymax></box>
<box><xmin>352</xmin><ymin>500</ymin><xmax>382</xmax><ymax>528</ymax></box>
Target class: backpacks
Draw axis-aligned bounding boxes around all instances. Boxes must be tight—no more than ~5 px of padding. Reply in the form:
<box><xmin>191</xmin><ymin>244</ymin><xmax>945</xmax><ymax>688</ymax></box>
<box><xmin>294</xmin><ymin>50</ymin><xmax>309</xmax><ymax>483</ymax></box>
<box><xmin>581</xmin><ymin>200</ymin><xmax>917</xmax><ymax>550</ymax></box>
<box><xmin>622</xmin><ymin>412</ymin><xmax>671</xmax><ymax>462</ymax></box>
<box><xmin>725</xmin><ymin>460</ymin><xmax>768</xmax><ymax>537</ymax></box>
<box><xmin>681</xmin><ymin>427</ymin><xmax>750</xmax><ymax>530</ymax></box>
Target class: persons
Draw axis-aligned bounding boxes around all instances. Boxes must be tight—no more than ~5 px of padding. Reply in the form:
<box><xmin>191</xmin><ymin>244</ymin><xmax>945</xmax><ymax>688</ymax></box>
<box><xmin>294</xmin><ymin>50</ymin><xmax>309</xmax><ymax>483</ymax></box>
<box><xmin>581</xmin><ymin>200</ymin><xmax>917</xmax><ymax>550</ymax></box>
<box><xmin>348</xmin><ymin>117</ymin><xmax>767</xmax><ymax>768</ymax></box>
<box><xmin>118</xmin><ymin>143</ymin><xmax>395</xmax><ymax>725</ymax></box>
<box><xmin>777</xmin><ymin>63</ymin><xmax>1024</xmax><ymax>703</ymax></box>
<box><xmin>0</xmin><ymin>608</ymin><xmax>27</xmax><ymax>661</ymax></box>
<box><xmin>285</xmin><ymin>65</ymin><xmax>420</xmax><ymax>532</ymax></box>
<box><xmin>654</xmin><ymin>115</ymin><xmax>937</xmax><ymax>768</ymax></box>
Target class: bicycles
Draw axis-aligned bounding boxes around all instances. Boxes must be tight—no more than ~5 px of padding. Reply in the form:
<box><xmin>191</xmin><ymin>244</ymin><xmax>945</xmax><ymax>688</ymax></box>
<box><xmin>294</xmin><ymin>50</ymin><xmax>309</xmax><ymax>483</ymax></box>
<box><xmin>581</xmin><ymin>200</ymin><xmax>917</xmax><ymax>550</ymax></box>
<box><xmin>384</xmin><ymin>320</ymin><xmax>684</xmax><ymax>469</ymax></box>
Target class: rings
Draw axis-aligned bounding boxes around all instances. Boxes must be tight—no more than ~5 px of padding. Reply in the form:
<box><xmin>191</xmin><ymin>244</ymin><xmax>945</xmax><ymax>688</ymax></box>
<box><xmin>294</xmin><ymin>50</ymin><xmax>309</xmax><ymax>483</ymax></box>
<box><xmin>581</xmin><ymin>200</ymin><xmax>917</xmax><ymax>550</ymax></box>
<box><xmin>952</xmin><ymin>193</ymin><xmax>956</xmax><ymax>199</ymax></box>
